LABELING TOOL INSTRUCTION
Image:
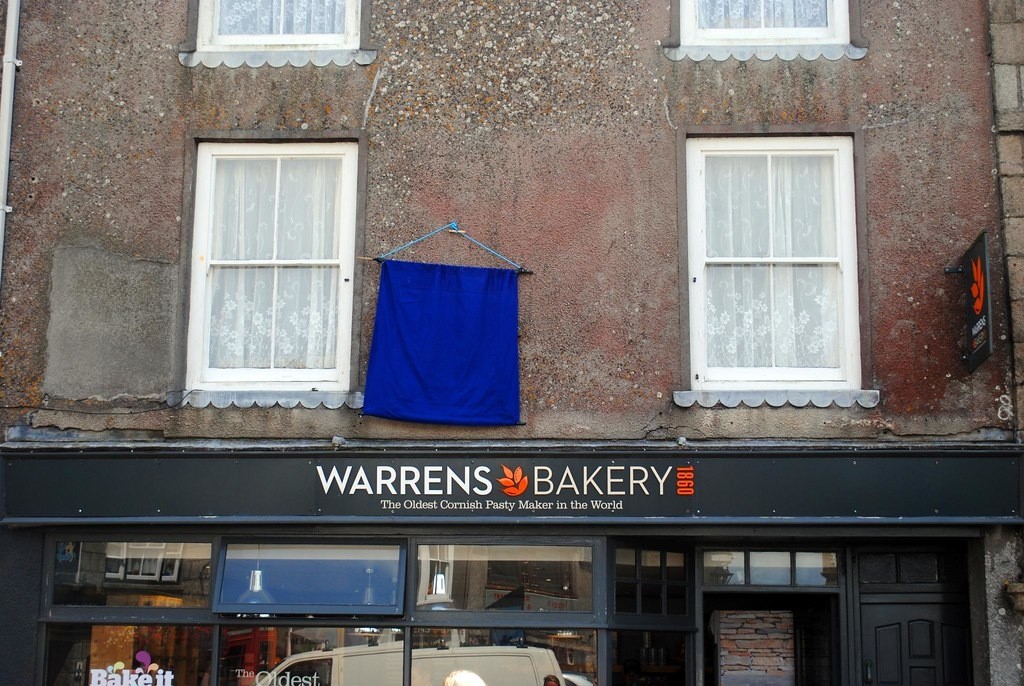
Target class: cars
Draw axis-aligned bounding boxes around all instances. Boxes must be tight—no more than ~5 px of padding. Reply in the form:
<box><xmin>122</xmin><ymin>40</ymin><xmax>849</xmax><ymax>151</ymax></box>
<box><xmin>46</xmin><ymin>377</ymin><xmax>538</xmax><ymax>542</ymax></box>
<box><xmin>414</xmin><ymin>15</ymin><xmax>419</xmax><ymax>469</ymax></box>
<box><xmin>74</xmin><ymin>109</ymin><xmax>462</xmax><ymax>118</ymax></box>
<box><xmin>563</xmin><ymin>672</ymin><xmax>594</xmax><ymax>686</ymax></box>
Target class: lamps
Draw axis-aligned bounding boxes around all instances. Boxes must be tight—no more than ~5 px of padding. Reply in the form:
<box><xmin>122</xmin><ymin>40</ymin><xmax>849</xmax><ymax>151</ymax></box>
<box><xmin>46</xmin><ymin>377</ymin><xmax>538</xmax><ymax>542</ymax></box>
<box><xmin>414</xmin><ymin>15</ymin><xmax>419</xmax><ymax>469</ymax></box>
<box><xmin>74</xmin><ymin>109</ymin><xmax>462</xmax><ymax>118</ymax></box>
<box><xmin>355</xmin><ymin>568</ymin><xmax>381</xmax><ymax>633</ymax></box>
<box><xmin>235</xmin><ymin>544</ymin><xmax>275</xmax><ymax>617</ymax></box>
<box><xmin>417</xmin><ymin>545</ymin><xmax>456</xmax><ymax>611</ymax></box>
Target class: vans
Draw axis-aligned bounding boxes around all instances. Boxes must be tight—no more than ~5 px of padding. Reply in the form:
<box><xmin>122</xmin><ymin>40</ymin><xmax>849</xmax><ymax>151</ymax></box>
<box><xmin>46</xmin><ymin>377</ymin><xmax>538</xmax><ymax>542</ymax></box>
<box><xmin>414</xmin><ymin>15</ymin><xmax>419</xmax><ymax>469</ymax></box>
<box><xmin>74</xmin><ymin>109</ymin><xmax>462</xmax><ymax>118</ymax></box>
<box><xmin>249</xmin><ymin>640</ymin><xmax>565</xmax><ymax>686</ymax></box>
